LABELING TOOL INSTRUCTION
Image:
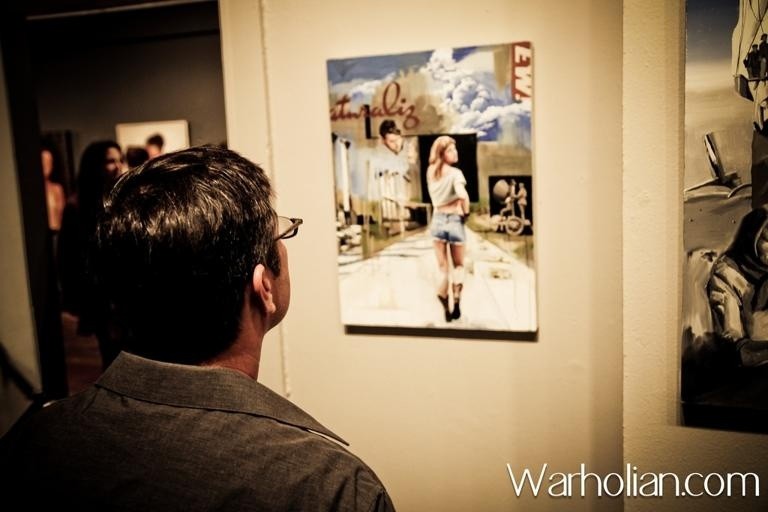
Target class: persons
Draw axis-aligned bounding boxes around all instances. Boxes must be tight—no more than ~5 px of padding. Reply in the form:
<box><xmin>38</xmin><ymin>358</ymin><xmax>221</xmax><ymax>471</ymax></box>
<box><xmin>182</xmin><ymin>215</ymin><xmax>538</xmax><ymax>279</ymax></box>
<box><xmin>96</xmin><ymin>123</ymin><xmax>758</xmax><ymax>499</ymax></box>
<box><xmin>500</xmin><ymin>179</ymin><xmax>528</xmax><ymax>221</ymax></box>
<box><xmin>0</xmin><ymin>139</ymin><xmax>396</xmax><ymax>512</ymax></box>
<box><xmin>370</xmin><ymin>120</ymin><xmax>417</xmax><ymax>179</ymax></box>
<box><xmin>146</xmin><ymin>135</ymin><xmax>164</xmax><ymax>158</ymax></box>
<box><xmin>748</xmin><ymin>34</ymin><xmax>768</xmax><ymax>78</ymax></box>
<box><xmin>426</xmin><ymin>136</ymin><xmax>470</xmax><ymax>322</ymax></box>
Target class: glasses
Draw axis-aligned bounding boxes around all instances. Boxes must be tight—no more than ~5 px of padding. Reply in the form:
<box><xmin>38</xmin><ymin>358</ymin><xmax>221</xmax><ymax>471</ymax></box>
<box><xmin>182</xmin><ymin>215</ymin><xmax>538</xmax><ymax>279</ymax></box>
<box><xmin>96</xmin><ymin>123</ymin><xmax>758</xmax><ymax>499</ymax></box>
<box><xmin>274</xmin><ymin>216</ymin><xmax>303</xmax><ymax>242</ymax></box>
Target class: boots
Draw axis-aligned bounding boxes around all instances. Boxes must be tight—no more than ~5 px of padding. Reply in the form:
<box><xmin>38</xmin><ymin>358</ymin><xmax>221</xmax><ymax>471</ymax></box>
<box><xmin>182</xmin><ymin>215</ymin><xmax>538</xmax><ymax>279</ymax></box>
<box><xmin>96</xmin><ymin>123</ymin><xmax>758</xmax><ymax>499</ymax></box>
<box><xmin>437</xmin><ymin>295</ymin><xmax>461</xmax><ymax>322</ymax></box>
